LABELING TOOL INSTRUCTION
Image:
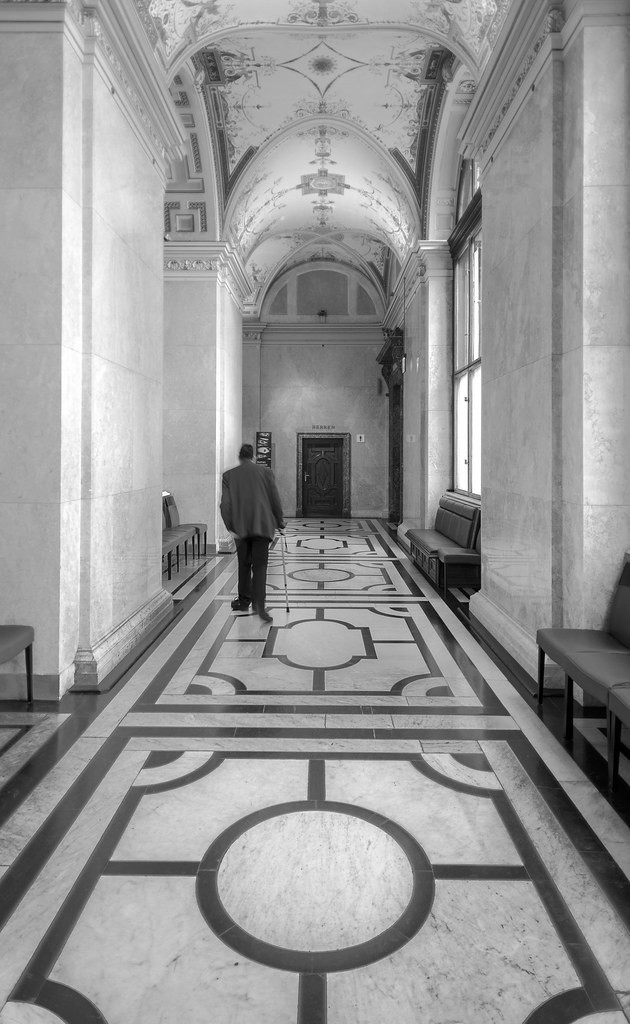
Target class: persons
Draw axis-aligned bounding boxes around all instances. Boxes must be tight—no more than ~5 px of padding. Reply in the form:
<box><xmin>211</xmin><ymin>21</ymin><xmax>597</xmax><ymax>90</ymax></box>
<box><xmin>219</xmin><ymin>444</ymin><xmax>284</xmax><ymax>611</ymax></box>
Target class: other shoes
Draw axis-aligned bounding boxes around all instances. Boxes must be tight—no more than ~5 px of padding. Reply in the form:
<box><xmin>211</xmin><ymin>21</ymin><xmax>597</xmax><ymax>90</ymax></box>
<box><xmin>231</xmin><ymin>597</ymin><xmax>249</xmax><ymax>610</ymax></box>
<box><xmin>252</xmin><ymin>602</ymin><xmax>264</xmax><ymax>611</ymax></box>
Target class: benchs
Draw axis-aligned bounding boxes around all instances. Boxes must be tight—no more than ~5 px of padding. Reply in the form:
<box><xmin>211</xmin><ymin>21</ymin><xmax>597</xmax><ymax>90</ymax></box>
<box><xmin>403</xmin><ymin>495</ymin><xmax>481</xmax><ymax>589</ymax></box>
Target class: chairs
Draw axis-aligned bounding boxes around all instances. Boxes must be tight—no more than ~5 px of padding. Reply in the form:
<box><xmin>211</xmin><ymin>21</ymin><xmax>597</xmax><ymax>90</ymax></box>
<box><xmin>437</xmin><ymin>526</ymin><xmax>481</xmax><ymax>601</ymax></box>
<box><xmin>0</xmin><ymin>625</ymin><xmax>35</xmax><ymax>705</ymax></box>
<box><xmin>162</xmin><ymin>495</ymin><xmax>208</xmax><ymax>581</ymax></box>
<box><xmin>536</xmin><ymin>551</ymin><xmax>630</xmax><ymax>794</ymax></box>
